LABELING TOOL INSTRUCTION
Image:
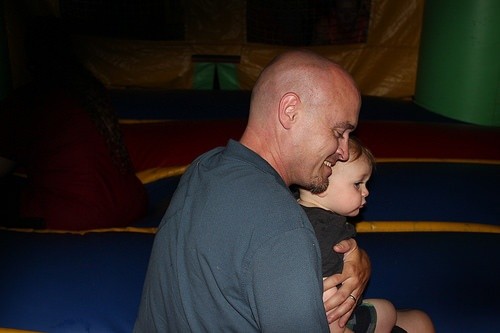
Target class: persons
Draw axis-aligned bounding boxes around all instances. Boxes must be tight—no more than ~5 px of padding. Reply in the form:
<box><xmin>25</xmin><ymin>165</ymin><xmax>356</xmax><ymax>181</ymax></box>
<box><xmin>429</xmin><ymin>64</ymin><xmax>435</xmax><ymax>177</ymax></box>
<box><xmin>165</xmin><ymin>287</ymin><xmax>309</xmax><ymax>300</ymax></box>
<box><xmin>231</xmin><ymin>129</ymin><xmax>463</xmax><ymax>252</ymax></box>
<box><xmin>297</xmin><ymin>138</ymin><xmax>397</xmax><ymax>333</ymax></box>
<box><xmin>132</xmin><ymin>48</ymin><xmax>435</xmax><ymax>333</ymax></box>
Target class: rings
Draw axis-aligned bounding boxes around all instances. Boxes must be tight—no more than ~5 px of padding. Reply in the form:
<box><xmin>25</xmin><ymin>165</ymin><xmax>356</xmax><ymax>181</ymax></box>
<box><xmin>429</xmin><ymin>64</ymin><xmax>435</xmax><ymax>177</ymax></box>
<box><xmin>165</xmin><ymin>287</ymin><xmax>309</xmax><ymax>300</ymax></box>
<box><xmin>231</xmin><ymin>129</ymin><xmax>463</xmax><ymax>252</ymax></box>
<box><xmin>350</xmin><ymin>294</ymin><xmax>356</xmax><ymax>303</ymax></box>
